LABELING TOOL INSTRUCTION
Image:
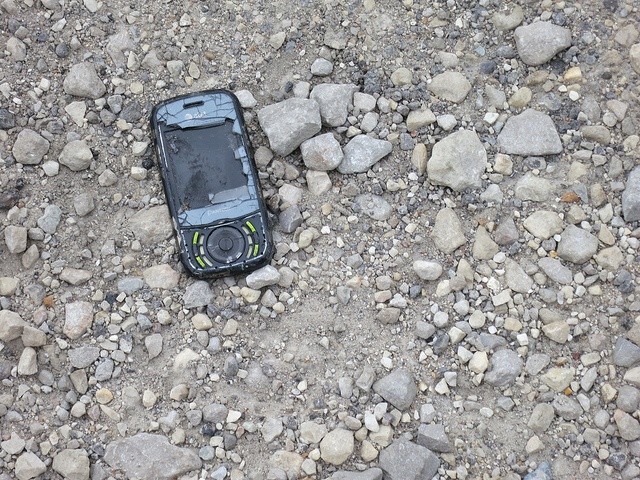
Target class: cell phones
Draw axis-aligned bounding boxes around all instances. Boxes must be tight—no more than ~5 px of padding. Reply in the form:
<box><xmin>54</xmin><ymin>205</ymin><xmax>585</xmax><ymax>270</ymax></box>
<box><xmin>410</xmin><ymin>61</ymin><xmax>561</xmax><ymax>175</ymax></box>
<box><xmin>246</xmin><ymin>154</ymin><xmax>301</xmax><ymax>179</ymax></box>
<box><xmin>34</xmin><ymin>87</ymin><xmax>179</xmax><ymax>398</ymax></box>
<box><xmin>151</xmin><ymin>88</ymin><xmax>274</xmax><ymax>279</ymax></box>
